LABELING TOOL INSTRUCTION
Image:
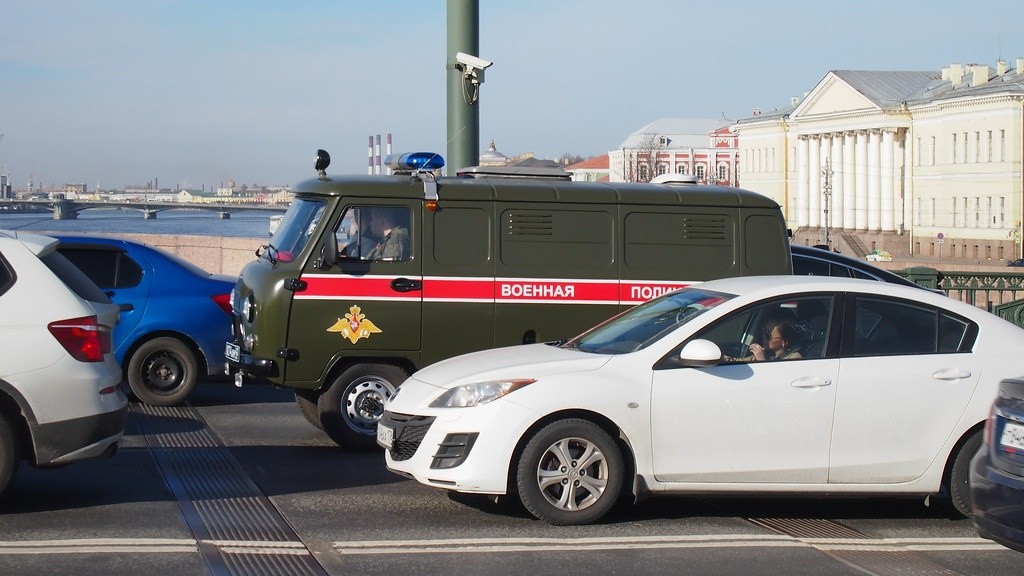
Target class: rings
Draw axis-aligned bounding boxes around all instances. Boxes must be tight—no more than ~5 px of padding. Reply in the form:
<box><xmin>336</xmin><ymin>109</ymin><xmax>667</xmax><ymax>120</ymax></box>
<box><xmin>755</xmin><ymin>345</ymin><xmax>759</xmax><ymax>348</ymax></box>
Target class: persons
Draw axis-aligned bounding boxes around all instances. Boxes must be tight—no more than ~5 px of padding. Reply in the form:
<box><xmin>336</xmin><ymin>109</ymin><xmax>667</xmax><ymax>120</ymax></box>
<box><xmin>339</xmin><ymin>206</ymin><xmax>410</xmax><ymax>261</ymax></box>
<box><xmin>340</xmin><ymin>207</ymin><xmax>378</xmax><ymax>260</ymax></box>
<box><xmin>871</xmin><ymin>240</ymin><xmax>876</xmax><ymax>252</ymax></box>
<box><xmin>719</xmin><ymin>312</ymin><xmax>804</xmax><ymax>364</ymax></box>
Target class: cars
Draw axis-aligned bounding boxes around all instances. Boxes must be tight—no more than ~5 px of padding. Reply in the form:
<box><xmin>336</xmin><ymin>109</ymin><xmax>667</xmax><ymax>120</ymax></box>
<box><xmin>377</xmin><ymin>274</ymin><xmax>1024</xmax><ymax>527</ymax></box>
<box><xmin>43</xmin><ymin>234</ymin><xmax>239</xmax><ymax>407</ymax></box>
<box><xmin>0</xmin><ymin>230</ymin><xmax>130</xmax><ymax>498</ymax></box>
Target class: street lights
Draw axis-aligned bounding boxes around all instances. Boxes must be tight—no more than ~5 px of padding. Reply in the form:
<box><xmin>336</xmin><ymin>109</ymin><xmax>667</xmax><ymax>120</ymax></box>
<box><xmin>819</xmin><ymin>157</ymin><xmax>834</xmax><ymax>245</ymax></box>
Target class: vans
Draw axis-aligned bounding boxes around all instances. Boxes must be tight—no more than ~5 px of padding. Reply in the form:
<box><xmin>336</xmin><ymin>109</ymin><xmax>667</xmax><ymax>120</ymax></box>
<box><xmin>224</xmin><ymin>149</ymin><xmax>794</xmax><ymax>452</ymax></box>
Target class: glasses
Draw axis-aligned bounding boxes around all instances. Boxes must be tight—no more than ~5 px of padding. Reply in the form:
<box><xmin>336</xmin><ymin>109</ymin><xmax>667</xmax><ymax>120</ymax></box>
<box><xmin>758</xmin><ymin>326</ymin><xmax>780</xmax><ymax>342</ymax></box>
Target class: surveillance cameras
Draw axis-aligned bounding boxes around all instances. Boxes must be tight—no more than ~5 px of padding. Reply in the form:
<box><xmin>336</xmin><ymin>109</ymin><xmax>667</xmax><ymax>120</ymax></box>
<box><xmin>455</xmin><ymin>52</ymin><xmax>494</xmax><ymax>77</ymax></box>
